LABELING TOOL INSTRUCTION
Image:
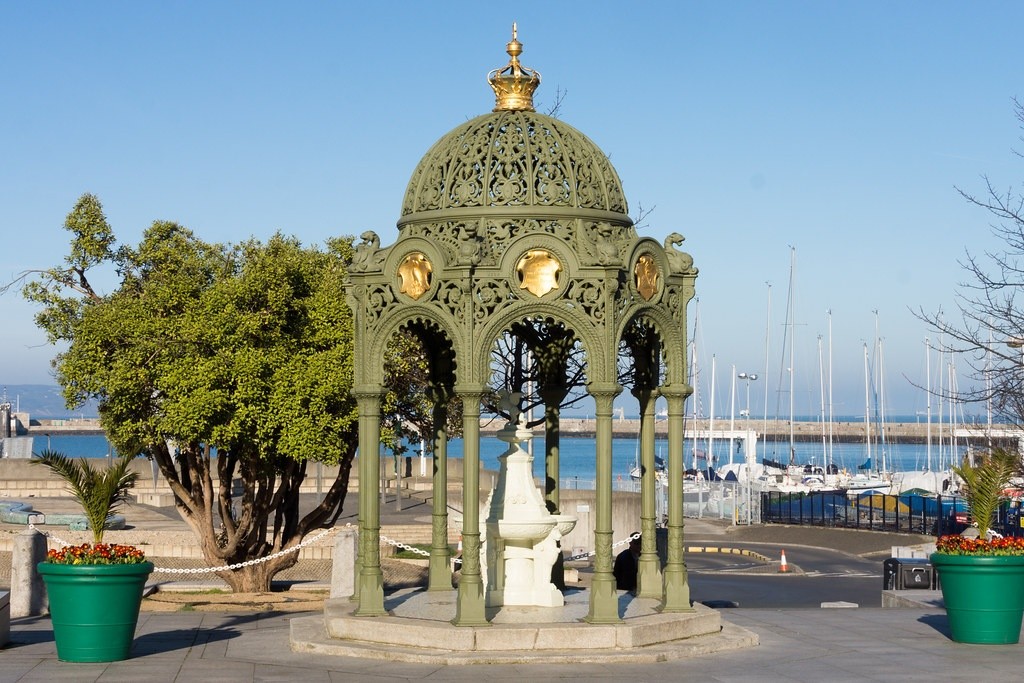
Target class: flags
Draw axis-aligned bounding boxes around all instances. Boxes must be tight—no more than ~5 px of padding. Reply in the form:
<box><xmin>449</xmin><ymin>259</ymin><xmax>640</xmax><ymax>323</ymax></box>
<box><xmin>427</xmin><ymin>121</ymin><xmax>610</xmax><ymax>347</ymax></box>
<box><xmin>655</xmin><ymin>455</ymin><xmax>664</xmax><ymax>464</ymax></box>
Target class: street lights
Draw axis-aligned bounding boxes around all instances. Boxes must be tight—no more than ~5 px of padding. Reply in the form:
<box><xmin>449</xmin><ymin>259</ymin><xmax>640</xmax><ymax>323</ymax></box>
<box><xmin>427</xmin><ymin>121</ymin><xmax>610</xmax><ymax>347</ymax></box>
<box><xmin>737</xmin><ymin>372</ymin><xmax>757</xmax><ymax>525</ymax></box>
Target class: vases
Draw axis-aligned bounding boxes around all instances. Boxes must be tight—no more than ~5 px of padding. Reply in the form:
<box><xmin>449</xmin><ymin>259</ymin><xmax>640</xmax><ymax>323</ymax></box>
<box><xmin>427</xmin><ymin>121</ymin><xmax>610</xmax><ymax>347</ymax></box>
<box><xmin>929</xmin><ymin>552</ymin><xmax>1024</xmax><ymax>646</ymax></box>
<box><xmin>35</xmin><ymin>560</ymin><xmax>154</xmax><ymax>665</ymax></box>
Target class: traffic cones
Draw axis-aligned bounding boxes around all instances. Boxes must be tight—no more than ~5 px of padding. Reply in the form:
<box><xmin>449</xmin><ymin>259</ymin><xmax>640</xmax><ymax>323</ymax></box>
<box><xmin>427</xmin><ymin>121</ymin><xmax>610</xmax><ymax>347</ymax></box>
<box><xmin>777</xmin><ymin>549</ymin><xmax>792</xmax><ymax>573</ymax></box>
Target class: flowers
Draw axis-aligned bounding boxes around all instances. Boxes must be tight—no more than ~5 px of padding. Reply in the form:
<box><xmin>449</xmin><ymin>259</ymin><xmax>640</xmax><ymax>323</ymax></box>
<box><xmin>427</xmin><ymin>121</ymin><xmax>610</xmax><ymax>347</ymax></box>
<box><xmin>935</xmin><ymin>533</ymin><xmax>1024</xmax><ymax>557</ymax></box>
<box><xmin>47</xmin><ymin>539</ymin><xmax>144</xmax><ymax>563</ymax></box>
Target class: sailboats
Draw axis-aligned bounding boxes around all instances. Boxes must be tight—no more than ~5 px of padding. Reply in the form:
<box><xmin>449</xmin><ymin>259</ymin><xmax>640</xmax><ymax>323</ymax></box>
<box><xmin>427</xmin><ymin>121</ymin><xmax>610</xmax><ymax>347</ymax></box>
<box><xmin>630</xmin><ymin>247</ymin><xmax>1023</xmax><ymax>516</ymax></box>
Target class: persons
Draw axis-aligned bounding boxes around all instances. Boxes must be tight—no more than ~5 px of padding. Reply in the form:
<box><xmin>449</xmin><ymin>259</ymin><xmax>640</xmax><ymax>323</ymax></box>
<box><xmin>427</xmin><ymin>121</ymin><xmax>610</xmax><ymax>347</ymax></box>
<box><xmin>613</xmin><ymin>532</ymin><xmax>642</xmax><ymax>591</ymax></box>
